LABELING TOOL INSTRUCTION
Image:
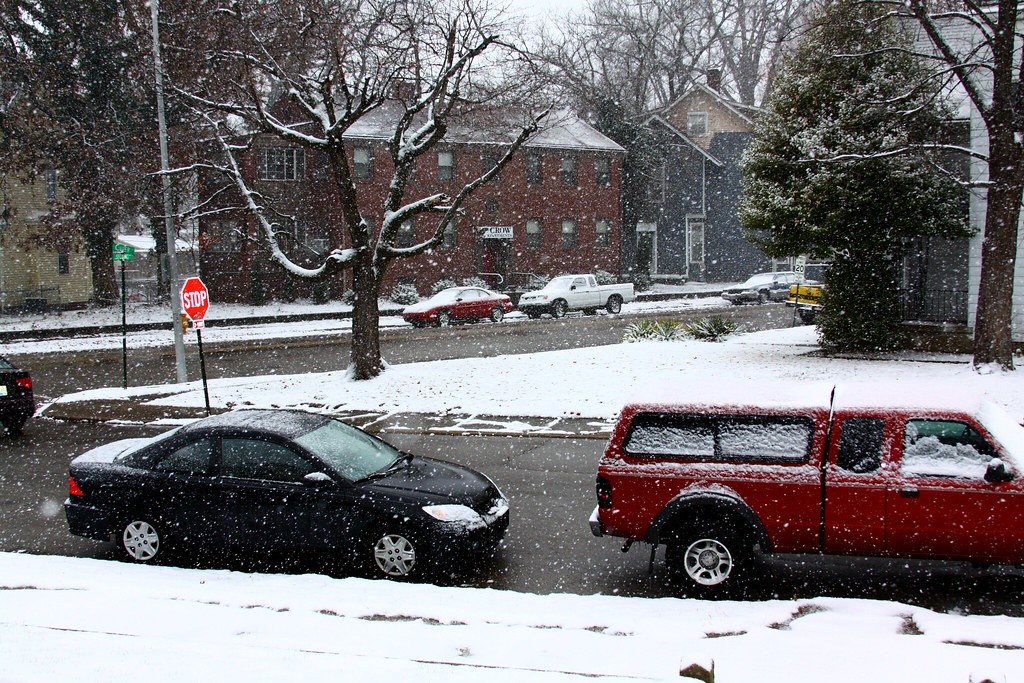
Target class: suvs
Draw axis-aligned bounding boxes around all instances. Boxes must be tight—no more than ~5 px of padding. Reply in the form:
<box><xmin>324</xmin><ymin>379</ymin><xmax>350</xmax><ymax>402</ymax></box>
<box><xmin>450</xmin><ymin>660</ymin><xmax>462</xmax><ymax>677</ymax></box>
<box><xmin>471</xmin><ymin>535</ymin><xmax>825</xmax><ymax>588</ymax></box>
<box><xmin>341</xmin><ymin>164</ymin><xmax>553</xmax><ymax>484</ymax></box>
<box><xmin>785</xmin><ymin>261</ymin><xmax>833</xmax><ymax>325</ymax></box>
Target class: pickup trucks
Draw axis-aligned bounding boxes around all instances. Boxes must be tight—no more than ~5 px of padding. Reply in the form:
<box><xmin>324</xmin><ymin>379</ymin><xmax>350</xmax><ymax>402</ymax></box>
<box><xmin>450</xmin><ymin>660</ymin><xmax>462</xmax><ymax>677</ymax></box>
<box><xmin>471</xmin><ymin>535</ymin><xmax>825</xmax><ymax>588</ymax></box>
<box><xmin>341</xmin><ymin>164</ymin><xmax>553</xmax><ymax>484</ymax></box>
<box><xmin>517</xmin><ymin>274</ymin><xmax>635</xmax><ymax>318</ymax></box>
<box><xmin>589</xmin><ymin>399</ymin><xmax>1023</xmax><ymax>592</ymax></box>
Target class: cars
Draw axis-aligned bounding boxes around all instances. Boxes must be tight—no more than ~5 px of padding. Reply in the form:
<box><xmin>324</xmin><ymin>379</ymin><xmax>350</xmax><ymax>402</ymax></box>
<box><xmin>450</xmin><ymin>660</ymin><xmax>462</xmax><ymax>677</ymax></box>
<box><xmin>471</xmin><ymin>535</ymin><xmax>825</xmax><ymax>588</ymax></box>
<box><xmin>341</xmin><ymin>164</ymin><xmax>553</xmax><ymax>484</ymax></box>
<box><xmin>64</xmin><ymin>409</ymin><xmax>511</xmax><ymax>576</ymax></box>
<box><xmin>721</xmin><ymin>271</ymin><xmax>806</xmax><ymax>305</ymax></box>
<box><xmin>0</xmin><ymin>356</ymin><xmax>35</xmax><ymax>433</ymax></box>
<box><xmin>402</xmin><ymin>287</ymin><xmax>514</xmax><ymax>327</ymax></box>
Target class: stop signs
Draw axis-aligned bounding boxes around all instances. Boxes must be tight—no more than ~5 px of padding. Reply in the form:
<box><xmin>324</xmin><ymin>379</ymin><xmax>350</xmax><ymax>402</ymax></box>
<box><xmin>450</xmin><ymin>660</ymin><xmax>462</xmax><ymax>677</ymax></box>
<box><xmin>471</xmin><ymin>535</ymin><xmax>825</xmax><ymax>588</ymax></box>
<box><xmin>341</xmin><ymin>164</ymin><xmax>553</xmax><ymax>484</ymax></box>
<box><xmin>180</xmin><ymin>277</ymin><xmax>210</xmax><ymax>330</ymax></box>
